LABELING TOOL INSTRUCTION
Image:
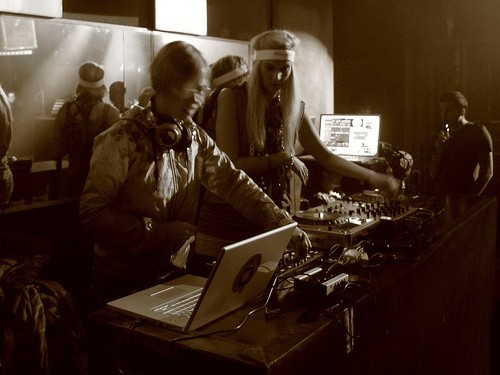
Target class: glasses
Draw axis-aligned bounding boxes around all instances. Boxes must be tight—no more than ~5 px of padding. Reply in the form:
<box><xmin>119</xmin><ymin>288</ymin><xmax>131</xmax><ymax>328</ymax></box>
<box><xmin>183</xmin><ymin>88</ymin><xmax>211</xmax><ymax>100</ymax></box>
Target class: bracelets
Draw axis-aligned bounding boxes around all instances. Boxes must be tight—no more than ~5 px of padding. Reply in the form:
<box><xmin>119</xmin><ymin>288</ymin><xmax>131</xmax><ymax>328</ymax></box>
<box><xmin>284</xmin><ymin>154</ymin><xmax>294</xmax><ymax>169</ymax></box>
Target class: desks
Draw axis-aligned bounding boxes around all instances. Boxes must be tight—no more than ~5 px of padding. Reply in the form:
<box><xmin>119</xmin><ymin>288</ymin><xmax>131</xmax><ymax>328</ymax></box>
<box><xmin>90</xmin><ymin>189</ymin><xmax>498</xmax><ymax>375</ymax></box>
<box><xmin>0</xmin><ymin>158</ymin><xmax>70</xmax><ymax>216</ymax></box>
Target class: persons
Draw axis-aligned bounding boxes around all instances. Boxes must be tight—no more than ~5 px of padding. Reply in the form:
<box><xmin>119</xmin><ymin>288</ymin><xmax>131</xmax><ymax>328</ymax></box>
<box><xmin>433</xmin><ymin>89</ymin><xmax>492</xmax><ymax>194</ymax></box>
<box><xmin>0</xmin><ymin>30</ymin><xmax>401</xmax><ymax>308</ymax></box>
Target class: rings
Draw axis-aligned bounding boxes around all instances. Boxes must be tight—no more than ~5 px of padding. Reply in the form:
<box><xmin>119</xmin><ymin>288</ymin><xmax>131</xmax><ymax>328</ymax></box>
<box><xmin>303</xmin><ymin>172</ymin><xmax>305</xmax><ymax>175</ymax></box>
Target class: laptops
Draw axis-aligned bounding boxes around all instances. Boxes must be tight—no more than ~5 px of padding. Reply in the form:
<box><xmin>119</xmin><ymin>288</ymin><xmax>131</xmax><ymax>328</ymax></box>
<box><xmin>297</xmin><ymin>113</ymin><xmax>382</xmax><ymax>164</ymax></box>
<box><xmin>106</xmin><ymin>221</ymin><xmax>298</xmax><ymax>333</ymax></box>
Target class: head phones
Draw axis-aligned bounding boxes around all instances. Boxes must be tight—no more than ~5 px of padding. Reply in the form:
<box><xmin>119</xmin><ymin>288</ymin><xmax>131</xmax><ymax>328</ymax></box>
<box><xmin>145</xmin><ymin>95</ymin><xmax>193</xmax><ymax>150</ymax></box>
